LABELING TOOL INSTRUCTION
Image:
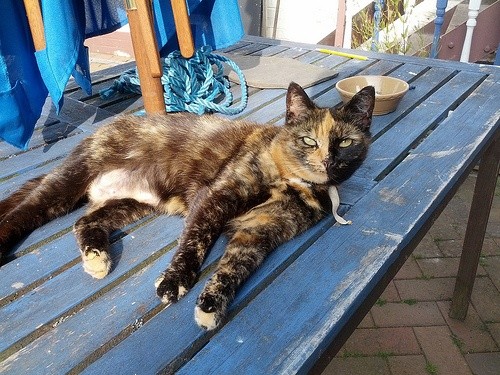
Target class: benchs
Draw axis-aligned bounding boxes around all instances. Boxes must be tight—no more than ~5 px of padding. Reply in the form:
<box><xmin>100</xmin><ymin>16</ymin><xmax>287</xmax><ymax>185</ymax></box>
<box><xmin>0</xmin><ymin>33</ymin><xmax>500</xmax><ymax>374</ymax></box>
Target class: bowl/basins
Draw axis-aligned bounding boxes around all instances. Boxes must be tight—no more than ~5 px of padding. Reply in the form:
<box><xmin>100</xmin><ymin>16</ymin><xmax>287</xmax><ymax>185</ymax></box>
<box><xmin>337</xmin><ymin>75</ymin><xmax>411</xmax><ymax>116</ymax></box>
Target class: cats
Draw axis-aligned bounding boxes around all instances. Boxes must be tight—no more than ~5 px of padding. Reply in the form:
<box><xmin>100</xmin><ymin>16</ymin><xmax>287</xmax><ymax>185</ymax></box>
<box><xmin>0</xmin><ymin>81</ymin><xmax>376</xmax><ymax>332</ymax></box>
